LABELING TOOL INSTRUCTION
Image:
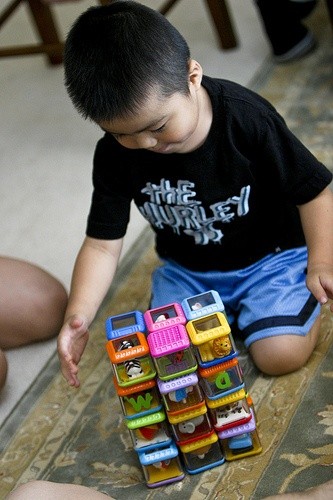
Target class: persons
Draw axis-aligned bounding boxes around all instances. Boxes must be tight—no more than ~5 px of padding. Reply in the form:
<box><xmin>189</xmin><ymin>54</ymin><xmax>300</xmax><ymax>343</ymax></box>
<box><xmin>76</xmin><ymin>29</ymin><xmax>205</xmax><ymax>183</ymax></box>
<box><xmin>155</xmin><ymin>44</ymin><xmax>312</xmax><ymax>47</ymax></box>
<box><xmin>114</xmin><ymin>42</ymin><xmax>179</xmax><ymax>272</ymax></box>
<box><xmin>0</xmin><ymin>256</ymin><xmax>68</xmax><ymax>392</ymax></box>
<box><xmin>56</xmin><ymin>0</ymin><xmax>333</xmax><ymax>390</ymax></box>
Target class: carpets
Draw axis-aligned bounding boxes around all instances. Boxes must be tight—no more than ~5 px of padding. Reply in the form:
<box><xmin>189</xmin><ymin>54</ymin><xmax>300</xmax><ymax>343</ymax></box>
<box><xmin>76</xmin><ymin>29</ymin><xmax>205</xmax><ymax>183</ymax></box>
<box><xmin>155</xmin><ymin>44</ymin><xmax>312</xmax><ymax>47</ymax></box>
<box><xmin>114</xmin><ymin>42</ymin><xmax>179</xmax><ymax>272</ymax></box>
<box><xmin>1</xmin><ymin>1</ymin><xmax>332</xmax><ymax>500</ymax></box>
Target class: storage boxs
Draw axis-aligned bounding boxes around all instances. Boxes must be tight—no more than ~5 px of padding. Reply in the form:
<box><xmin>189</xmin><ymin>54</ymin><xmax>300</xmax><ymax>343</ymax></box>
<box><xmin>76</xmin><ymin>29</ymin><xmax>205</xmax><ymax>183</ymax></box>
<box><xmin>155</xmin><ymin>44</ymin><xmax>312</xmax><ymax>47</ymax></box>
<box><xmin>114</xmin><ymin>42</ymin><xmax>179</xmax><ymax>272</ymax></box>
<box><xmin>105</xmin><ymin>289</ymin><xmax>263</xmax><ymax>489</ymax></box>
